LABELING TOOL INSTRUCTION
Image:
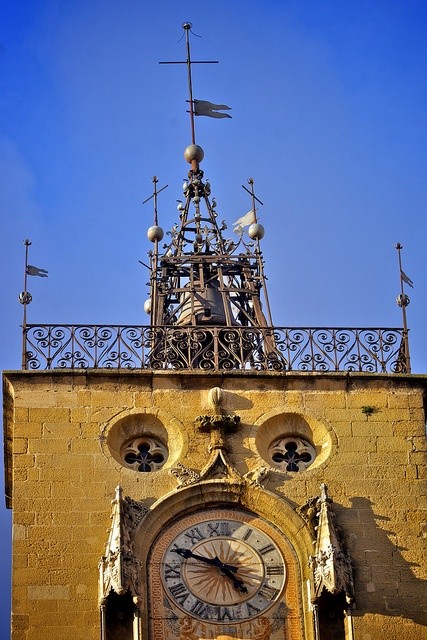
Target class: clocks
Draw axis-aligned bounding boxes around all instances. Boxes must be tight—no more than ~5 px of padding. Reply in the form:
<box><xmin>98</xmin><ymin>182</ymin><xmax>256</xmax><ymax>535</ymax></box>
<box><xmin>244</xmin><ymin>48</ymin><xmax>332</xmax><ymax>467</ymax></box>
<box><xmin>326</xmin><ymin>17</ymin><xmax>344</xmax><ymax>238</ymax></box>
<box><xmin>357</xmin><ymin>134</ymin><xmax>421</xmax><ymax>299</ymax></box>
<box><xmin>158</xmin><ymin>519</ymin><xmax>286</xmax><ymax>626</ymax></box>
